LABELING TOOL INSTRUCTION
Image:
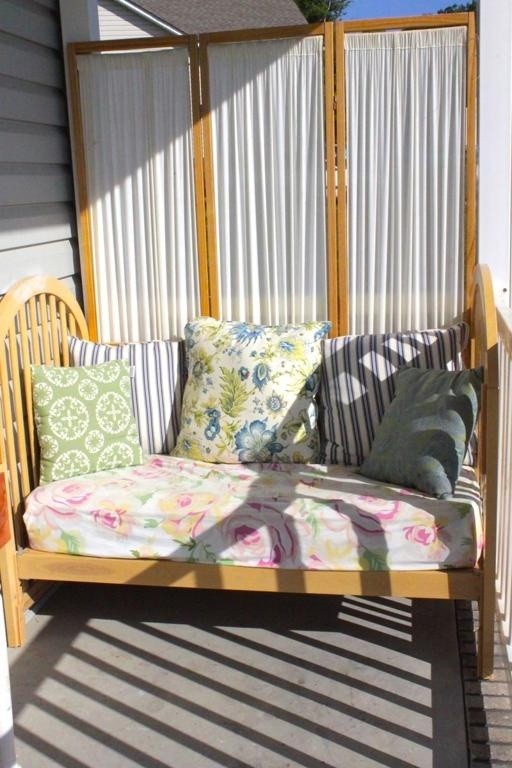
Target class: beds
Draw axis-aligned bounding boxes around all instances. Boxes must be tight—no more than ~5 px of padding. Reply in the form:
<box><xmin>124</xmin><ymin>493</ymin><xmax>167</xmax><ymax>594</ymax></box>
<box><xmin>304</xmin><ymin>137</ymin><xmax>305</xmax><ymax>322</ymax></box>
<box><xmin>0</xmin><ymin>262</ymin><xmax>503</xmax><ymax>680</ymax></box>
